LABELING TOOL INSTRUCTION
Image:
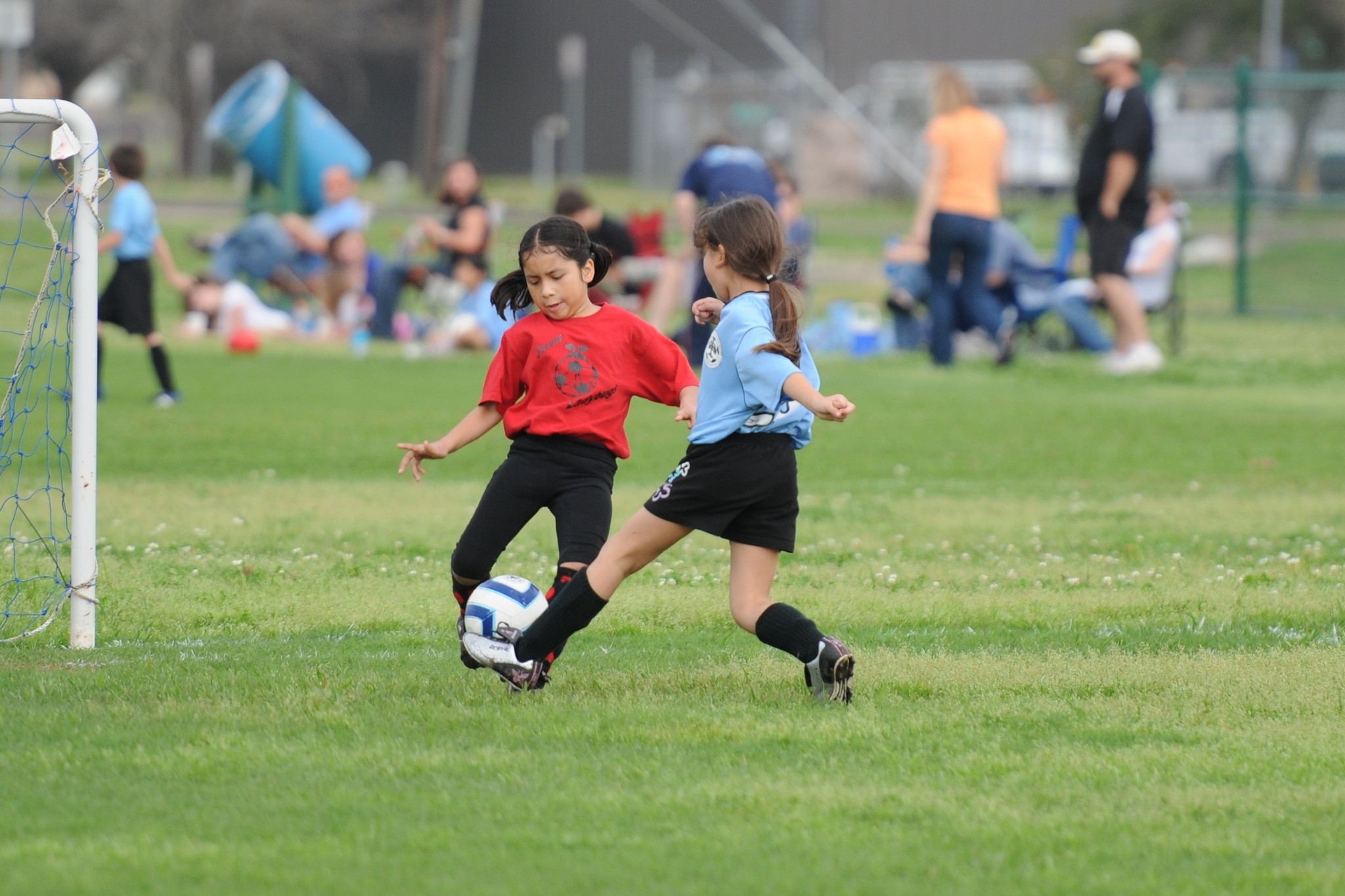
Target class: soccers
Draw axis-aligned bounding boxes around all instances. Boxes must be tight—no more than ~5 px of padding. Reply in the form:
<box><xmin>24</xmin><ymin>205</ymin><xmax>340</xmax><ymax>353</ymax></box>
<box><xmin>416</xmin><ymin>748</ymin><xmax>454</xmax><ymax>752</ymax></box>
<box><xmin>463</xmin><ymin>573</ymin><xmax>551</xmax><ymax>646</ymax></box>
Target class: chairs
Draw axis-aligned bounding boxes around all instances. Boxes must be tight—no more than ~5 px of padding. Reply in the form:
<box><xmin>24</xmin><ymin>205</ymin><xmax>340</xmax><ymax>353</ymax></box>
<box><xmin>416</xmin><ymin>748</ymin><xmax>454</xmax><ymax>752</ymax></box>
<box><xmin>1009</xmin><ymin>210</ymin><xmax>1084</xmax><ymax>353</ymax></box>
<box><xmin>1145</xmin><ymin>248</ymin><xmax>1184</xmax><ymax>358</ymax></box>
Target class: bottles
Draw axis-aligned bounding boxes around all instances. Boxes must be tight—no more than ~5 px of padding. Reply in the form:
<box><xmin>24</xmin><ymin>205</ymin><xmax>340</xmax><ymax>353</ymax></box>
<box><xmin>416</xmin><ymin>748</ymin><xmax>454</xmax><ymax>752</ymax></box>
<box><xmin>849</xmin><ymin>302</ymin><xmax>882</xmax><ymax>355</ymax></box>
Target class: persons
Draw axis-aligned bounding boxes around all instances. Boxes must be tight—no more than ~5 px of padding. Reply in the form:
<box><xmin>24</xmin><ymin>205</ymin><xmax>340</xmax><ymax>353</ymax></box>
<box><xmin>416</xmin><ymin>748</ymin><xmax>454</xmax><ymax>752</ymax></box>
<box><xmin>465</xmin><ymin>192</ymin><xmax>858</xmax><ymax>706</ymax></box>
<box><xmin>63</xmin><ymin>141</ymin><xmax>198</xmax><ymax>406</ymax></box>
<box><xmin>183</xmin><ymin>28</ymin><xmax>1190</xmax><ymax>374</ymax></box>
<box><xmin>398</xmin><ymin>216</ymin><xmax>699</xmax><ymax>689</ymax></box>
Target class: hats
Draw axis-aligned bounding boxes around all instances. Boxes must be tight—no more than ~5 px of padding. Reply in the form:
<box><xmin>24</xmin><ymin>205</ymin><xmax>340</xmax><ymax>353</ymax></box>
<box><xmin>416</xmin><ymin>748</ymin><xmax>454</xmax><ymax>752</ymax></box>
<box><xmin>1075</xmin><ymin>29</ymin><xmax>1143</xmax><ymax>65</ymax></box>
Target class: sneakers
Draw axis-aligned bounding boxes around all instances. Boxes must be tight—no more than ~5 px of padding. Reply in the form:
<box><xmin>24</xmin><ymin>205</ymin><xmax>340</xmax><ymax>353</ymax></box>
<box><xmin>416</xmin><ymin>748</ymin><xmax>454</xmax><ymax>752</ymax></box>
<box><xmin>804</xmin><ymin>634</ymin><xmax>856</xmax><ymax>707</ymax></box>
<box><xmin>152</xmin><ymin>392</ymin><xmax>179</xmax><ymax>408</ymax></box>
<box><xmin>1106</xmin><ymin>338</ymin><xmax>1164</xmax><ymax>374</ymax></box>
<box><xmin>992</xmin><ymin>303</ymin><xmax>1019</xmax><ymax>364</ymax></box>
<box><xmin>456</xmin><ymin>615</ymin><xmax>485</xmax><ymax>670</ymax></box>
<box><xmin>462</xmin><ymin>630</ymin><xmax>544</xmax><ymax>690</ymax></box>
